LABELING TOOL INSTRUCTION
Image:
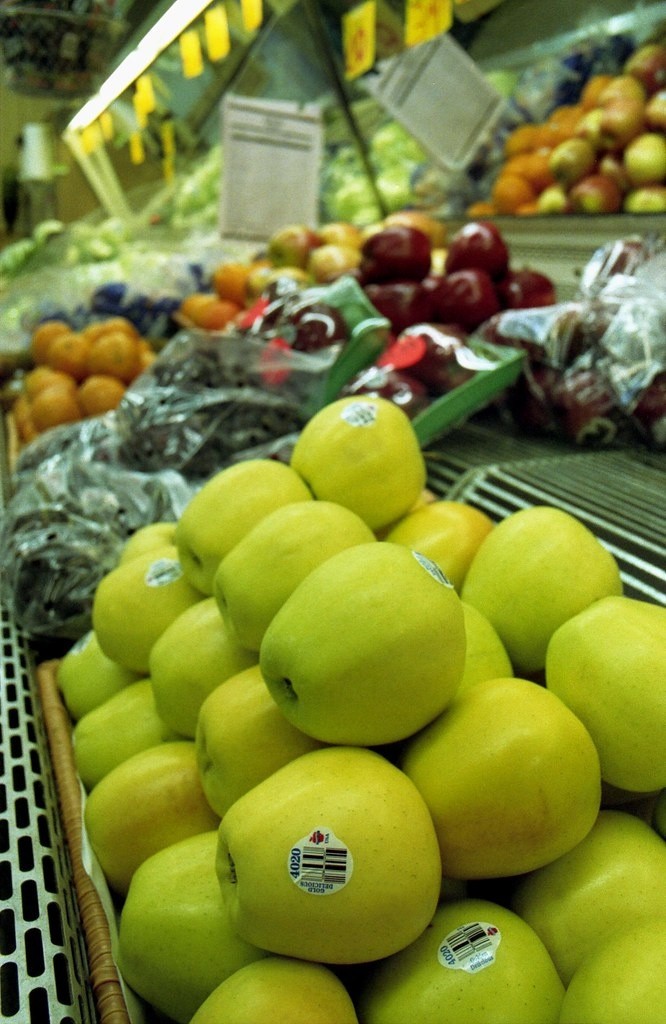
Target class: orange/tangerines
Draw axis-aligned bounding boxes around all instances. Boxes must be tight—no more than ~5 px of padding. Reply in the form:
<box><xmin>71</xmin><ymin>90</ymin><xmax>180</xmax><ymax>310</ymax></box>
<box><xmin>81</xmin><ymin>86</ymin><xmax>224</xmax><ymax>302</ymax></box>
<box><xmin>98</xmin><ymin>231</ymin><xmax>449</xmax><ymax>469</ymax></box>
<box><xmin>16</xmin><ymin>317</ymin><xmax>151</xmax><ymax>442</ymax></box>
<box><xmin>464</xmin><ymin>74</ymin><xmax>611</xmax><ymax>216</ymax></box>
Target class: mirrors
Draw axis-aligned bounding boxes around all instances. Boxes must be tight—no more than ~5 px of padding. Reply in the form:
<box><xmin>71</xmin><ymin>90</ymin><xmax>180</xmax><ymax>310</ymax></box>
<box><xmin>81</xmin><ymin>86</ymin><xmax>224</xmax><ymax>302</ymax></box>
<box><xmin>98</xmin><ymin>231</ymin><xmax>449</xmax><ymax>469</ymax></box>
<box><xmin>79</xmin><ymin>0</ymin><xmax>666</xmax><ymax>226</ymax></box>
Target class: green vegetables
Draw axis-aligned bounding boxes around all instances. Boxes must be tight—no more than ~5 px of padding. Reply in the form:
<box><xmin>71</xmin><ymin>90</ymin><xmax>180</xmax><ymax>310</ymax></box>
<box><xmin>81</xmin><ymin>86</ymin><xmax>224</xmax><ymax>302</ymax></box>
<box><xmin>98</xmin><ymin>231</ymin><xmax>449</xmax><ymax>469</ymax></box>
<box><xmin>0</xmin><ymin>330</ymin><xmax>307</xmax><ymax>643</ymax></box>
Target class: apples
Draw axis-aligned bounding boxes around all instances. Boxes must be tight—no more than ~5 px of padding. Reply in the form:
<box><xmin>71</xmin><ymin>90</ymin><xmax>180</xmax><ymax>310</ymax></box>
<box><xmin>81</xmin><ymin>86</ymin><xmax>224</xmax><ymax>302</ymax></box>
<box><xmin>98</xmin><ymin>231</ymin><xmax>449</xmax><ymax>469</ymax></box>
<box><xmin>56</xmin><ymin>397</ymin><xmax>666</xmax><ymax>1024</ymax></box>
<box><xmin>538</xmin><ymin>41</ymin><xmax>666</xmax><ymax>214</ymax></box>
<box><xmin>188</xmin><ymin>210</ymin><xmax>666</xmax><ymax>444</ymax></box>
